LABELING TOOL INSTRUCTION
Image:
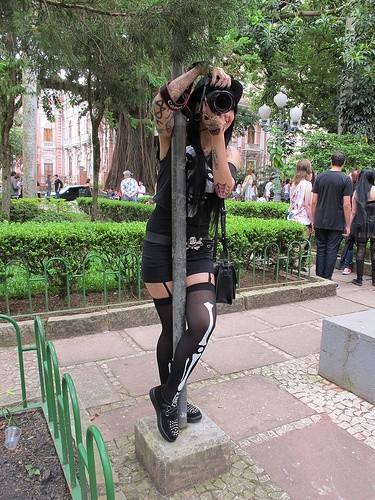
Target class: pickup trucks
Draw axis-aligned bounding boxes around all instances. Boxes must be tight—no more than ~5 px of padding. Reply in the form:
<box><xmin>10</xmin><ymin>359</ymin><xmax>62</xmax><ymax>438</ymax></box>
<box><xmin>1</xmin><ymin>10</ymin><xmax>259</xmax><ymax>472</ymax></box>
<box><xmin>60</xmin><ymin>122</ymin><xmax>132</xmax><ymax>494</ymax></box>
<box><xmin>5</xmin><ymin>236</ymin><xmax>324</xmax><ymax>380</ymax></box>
<box><xmin>44</xmin><ymin>185</ymin><xmax>108</xmax><ymax>201</ymax></box>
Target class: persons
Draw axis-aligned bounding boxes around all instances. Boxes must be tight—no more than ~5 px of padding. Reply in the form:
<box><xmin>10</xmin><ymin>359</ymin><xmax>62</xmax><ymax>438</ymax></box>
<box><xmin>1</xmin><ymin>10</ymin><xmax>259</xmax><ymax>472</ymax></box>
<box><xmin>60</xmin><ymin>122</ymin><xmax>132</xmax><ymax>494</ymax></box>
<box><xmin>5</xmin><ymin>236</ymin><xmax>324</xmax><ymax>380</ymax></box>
<box><xmin>311</xmin><ymin>151</ymin><xmax>352</xmax><ymax>289</ymax></box>
<box><xmin>85</xmin><ymin>170</ymin><xmax>145</xmax><ymax>203</ymax></box>
<box><xmin>234</xmin><ymin>159</ymin><xmax>375</xmax><ymax>287</ymax></box>
<box><xmin>10</xmin><ymin>170</ymin><xmax>41</xmax><ymax>200</ymax></box>
<box><xmin>44</xmin><ymin>174</ymin><xmax>62</xmax><ymax>201</ymax></box>
<box><xmin>141</xmin><ymin>63</ymin><xmax>243</xmax><ymax>443</ymax></box>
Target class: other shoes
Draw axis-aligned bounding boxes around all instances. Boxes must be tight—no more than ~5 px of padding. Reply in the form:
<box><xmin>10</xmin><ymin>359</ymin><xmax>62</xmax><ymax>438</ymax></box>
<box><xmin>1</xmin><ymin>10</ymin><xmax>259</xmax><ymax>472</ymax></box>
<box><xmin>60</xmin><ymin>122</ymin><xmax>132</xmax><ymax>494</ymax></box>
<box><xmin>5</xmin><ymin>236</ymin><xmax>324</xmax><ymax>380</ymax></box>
<box><xmin>353</xmin><ymin>278</ymin><xmax>362</xmax><ymax>286</ymax></box>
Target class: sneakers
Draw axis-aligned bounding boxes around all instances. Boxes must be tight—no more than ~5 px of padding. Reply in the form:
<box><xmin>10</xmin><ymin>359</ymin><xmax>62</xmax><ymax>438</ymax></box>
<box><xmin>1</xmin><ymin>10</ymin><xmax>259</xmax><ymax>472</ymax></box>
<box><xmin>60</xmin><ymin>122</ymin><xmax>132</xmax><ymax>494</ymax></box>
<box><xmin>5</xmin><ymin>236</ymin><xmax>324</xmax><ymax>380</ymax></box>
<box><xmin>343</xmin><ymin>267</ymin><xmax>351</xmax><ymax>274</ymax></box>
<box><xmin>149</xmin><ymin>385</ymin><xmax>179</xmax><ymax>441</ymax></box>
<box><xmin>185</xmin><ymin>401</ymin><xmax>203</xmax><ymax>423</ymax></box>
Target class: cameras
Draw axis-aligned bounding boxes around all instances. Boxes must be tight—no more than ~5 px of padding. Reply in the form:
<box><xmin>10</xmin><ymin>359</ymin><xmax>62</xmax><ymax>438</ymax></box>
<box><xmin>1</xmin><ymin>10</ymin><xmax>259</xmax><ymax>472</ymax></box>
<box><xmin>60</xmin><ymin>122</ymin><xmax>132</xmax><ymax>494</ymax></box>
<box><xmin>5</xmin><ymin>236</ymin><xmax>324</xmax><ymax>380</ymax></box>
<box><xmin>196</xmin><ymin>75</ymin><xmax>234</xmax><ymax>120</ymax></box>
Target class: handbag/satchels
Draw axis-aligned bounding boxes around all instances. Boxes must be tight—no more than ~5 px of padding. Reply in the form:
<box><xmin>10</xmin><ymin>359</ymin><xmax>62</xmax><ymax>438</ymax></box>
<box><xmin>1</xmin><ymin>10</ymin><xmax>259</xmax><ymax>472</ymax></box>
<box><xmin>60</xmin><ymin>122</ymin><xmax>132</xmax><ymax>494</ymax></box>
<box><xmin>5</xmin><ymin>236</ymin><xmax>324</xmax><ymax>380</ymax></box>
<box><xmin>214</xmin><ymin>260</ymin><xmax>238</xmax><ymax>305</ymax></box>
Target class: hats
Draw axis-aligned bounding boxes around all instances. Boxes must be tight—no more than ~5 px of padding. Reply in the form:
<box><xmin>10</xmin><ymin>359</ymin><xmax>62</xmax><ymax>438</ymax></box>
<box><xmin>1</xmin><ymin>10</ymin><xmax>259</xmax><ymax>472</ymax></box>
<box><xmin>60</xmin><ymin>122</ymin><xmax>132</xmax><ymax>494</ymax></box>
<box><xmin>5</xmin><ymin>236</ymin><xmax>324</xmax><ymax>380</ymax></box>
<box><xmin>182</xmin><ymin>76</ymin><xmax>243</xmax><ymax>119</ymax></box>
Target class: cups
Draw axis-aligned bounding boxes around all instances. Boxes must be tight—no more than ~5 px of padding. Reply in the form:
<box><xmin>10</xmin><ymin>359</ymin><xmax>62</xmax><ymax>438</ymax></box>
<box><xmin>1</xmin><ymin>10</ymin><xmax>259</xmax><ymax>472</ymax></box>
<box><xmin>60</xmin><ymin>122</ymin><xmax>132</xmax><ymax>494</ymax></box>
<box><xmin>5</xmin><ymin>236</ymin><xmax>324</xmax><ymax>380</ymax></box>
<box><xmin>5</xmin><ymin>427</ymin><xmax>21</xmax><ymax>450</ymax></box>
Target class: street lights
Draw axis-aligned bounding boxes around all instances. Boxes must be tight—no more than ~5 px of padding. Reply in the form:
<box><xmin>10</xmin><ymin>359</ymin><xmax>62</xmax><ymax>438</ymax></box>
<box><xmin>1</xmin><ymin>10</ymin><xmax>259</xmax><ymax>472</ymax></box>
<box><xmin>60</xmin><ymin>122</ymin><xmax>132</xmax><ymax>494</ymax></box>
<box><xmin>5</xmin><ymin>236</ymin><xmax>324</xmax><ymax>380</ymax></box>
<box><xmin>258</xmin><ymin>92</ymin><xmax>302</xmax><ymax>201</ymax></box>
